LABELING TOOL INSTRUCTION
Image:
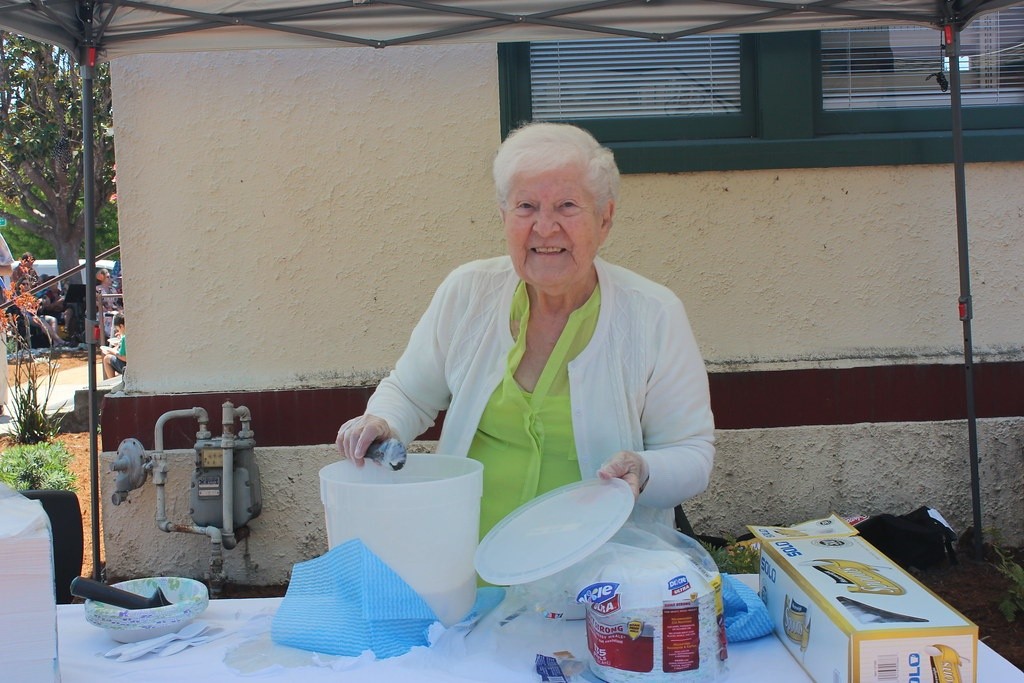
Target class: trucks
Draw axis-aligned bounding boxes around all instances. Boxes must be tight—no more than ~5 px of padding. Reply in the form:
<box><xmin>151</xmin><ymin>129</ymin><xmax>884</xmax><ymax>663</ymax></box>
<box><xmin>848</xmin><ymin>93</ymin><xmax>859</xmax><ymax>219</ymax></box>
<box><xmin>4</xmin><ymin>259</ymin><xmax>116</xmax><ymax>291</ymax></box>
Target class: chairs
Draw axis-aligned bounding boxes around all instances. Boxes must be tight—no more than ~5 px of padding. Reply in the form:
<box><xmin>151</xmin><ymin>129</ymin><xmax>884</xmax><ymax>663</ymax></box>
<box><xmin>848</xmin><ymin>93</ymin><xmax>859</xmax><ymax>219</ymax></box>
<box><xmin>19</xmin><ymin>489</ymin><xmax>84</xmax><ymax>604</ymax></box>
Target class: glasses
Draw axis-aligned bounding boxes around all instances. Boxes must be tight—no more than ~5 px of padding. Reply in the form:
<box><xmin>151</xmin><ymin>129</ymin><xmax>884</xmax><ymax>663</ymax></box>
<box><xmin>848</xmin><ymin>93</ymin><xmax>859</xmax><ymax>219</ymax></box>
<box><xmin>22</xmin><ymin>283</ymin><xmax>28</xmax><ymax>286</ymax></box>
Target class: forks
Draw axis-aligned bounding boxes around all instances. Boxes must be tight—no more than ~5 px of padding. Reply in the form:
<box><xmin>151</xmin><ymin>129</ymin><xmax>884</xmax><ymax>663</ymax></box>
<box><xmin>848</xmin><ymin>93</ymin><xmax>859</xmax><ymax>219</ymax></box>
<box><xmin>105</xmin><ymin>622</ymin><xmax>225</xmax><ymax>662</ymax></box>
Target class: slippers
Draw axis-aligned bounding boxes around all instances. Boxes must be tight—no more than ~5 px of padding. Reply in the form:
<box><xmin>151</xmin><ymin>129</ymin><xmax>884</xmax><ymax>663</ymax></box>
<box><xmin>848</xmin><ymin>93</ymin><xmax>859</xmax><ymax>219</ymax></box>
<box><xmin>52</xmin><ymin>340</ymin><xmax>71</xmax><ymax>347</ymax></box>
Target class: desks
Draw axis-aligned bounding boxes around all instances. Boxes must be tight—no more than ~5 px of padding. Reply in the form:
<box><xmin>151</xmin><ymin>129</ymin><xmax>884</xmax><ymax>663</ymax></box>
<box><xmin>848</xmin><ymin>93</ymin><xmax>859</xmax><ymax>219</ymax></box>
<box><xmin>57</xmin><ymin>573</ymin><xmax>1024</xmax><ymax>683</ymax></box>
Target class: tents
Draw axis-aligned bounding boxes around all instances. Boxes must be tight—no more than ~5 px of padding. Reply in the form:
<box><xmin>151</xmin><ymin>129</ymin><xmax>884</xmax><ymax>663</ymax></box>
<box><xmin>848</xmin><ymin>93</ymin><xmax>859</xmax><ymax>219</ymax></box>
<box><xmin>1</xmin><ymin>0</ymin><xmax>1024</xmax><ymax>581</ymax></box>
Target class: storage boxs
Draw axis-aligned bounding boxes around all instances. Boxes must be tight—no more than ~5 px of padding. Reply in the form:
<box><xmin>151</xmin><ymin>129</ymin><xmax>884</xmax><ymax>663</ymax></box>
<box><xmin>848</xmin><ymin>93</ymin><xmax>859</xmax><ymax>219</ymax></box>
<box><xmin>745</xmin><ymin>512</ymin><xmax>979</xmax><ymax>683</ymax></box>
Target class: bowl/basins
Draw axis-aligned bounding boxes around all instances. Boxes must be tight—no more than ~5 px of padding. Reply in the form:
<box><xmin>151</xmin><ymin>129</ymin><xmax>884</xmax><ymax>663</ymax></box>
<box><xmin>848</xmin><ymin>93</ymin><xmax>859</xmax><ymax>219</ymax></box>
<box><xmin>100</xmin><ymin>345</ymin><xmax>117</xmax><ymax>355</ymax></box>
<box><xmin>107</xmin><ymin>338</ymin><xmax>119</xmax><ymax>346</ymax></box>
<box><xmin>84</xmin><ymin>577</ymin><xmax>208</xmax><ymax>643</ymax></box>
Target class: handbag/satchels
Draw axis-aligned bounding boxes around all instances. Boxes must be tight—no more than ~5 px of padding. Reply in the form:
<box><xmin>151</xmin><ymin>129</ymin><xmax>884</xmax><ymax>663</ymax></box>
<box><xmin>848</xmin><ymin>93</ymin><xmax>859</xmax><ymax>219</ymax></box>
<box><xmin>851</xmin><ymin>505</ymin><xmax>957</xmax><ymax>573</ymax></box>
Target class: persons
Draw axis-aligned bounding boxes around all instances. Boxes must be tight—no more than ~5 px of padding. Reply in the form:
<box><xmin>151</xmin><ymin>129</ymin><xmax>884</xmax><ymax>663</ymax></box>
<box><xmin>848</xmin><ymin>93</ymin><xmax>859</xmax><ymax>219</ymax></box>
<box><xmin>333</xmin><ymin>123</ymin><xmax>716</xmax><ymax>586</ymax></box>
<box><xmin>7</xmin><ymin>254</ymin><xmax>126</xmax><ymax>380</ymax></box>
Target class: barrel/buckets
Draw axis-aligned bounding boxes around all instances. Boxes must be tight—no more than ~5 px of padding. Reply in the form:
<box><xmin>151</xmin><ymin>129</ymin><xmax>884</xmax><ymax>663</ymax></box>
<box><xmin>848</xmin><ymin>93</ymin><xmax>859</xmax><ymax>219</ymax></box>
<box><xmin>318</xmin><ymin>455</ymin><xmax>484</xmax><ymax>630</ymax></box>
